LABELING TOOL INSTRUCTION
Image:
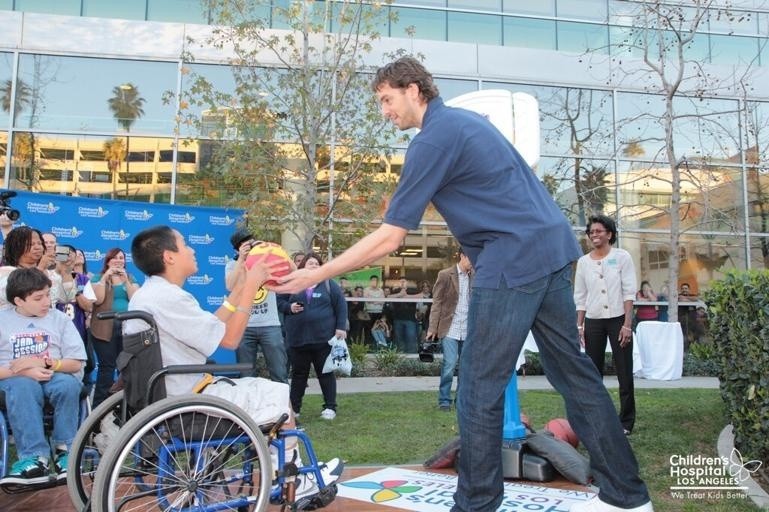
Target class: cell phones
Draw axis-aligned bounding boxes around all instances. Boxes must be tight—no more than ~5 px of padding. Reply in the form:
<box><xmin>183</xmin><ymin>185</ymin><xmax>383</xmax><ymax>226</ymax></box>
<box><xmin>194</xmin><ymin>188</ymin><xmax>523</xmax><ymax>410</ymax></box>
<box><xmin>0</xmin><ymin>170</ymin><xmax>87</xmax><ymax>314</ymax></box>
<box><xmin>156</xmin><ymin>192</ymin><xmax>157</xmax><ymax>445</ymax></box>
<box><xmin>297</xmin><ymin>301</ymin><xmax>304</xmax><ymax>307</ymax></box>
<box><xmin>53</xmin><ymin>245</ymin><xmax>70</xmax><ymax>262</ymax></box>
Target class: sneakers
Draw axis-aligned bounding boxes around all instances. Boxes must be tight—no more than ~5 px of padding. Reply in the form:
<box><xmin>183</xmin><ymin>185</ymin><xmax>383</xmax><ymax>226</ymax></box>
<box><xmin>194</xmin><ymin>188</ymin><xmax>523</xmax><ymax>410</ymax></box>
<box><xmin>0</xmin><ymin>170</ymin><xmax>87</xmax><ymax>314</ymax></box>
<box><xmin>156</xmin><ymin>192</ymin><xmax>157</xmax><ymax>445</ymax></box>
<box><xmin>569</xmin><ymin>496</ymin><xmax>655</xmax><ymax>512</ymax></box>
<box><xmin>7</xmin><ymin>454</ymin><xmax>52</xmax><ymax>477</ymax></box>
<box><xmin>320</xmin><ymin>409</ymin><xmax>336</xmax><ymax>421</ymax></box>
<box><xmin>269</xmin><ymin>457</ymin><xmax>344</xmax><ymax>512</ymax></box>
<box><xmin>440</xmin><ymin>405</ymin><xmax>451</xmax><ymax>412</ymax></box>
<box><xmin>54</xmin><ymin>447</ymin><xmax>84</xmax><ymax>482</ymax></box>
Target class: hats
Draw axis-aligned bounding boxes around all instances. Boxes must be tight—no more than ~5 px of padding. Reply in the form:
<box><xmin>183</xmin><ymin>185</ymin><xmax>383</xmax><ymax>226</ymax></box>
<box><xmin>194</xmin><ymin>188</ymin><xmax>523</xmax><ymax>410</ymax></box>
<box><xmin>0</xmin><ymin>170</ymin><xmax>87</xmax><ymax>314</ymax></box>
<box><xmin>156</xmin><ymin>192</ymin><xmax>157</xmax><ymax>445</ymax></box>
<box><xmin>230</xmin><ymin>229</ymin><xmax>254</xmax><ymax>249</ymax></box>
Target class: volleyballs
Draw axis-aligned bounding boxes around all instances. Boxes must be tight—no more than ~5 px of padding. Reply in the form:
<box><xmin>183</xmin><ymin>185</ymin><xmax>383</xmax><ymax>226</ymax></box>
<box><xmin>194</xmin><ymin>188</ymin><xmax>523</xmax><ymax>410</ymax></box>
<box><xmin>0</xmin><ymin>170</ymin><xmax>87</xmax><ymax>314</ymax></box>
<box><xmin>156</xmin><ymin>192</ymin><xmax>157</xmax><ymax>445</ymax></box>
<box><xmin>246</xmin><ymin>241</ymin><xmax>295</xmax><ymax>285</ymax></box>
<box><xmin>546</xmin><ymin>418</ymin><xmax>578</xmax><ymax>449</ymax></box>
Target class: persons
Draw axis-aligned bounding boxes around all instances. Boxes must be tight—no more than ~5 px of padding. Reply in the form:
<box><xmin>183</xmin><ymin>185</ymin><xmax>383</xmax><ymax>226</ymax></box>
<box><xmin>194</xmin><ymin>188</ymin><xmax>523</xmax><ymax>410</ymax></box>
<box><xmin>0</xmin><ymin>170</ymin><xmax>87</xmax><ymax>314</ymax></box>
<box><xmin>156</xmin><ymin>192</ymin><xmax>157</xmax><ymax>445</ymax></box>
<box><xmin>1</xmin><ymin>206</ymin><xmax>139</xmax><ymax>493</ymax></box>
<box><xmin>262</xmin><ymin>56</ymin><xmax>655</xmax><ymax>512</ymax></box>
<box><xmin>225</xmin><ymin>229</ymin><xmax>289</xmax><ymax>385</ymax></box>
<box><xmin>123</xmin><ymin>225</ymin><xmax>344</xmax><ymax>512</ymax></box>
<box><xmin>337</xmin><ymin>276</ymin><xmax>434</xmax><ymax>355</ymax></box>
<box><xmin>573</xmin><ymin>216</ymin><xmax>638</xmax><ymax>435</ymax></box>
<box><xmin>425</xmin><ymin>246</ymin><xmax>475</xmax><ymax>412</ymax></box>
<box><xmin>279</xmin><ymin>251</ymin><xmax>309</xmax><ymax>387</ymax></box>
<box><xmin>636</xmin><ymin>281</ymin><xmax>710</xmax><ymax>352</ymax></box>
<box><xmin>276</xmin><ymin>253</ymin><xmax>348</xmax><ymax>420</ymax></box>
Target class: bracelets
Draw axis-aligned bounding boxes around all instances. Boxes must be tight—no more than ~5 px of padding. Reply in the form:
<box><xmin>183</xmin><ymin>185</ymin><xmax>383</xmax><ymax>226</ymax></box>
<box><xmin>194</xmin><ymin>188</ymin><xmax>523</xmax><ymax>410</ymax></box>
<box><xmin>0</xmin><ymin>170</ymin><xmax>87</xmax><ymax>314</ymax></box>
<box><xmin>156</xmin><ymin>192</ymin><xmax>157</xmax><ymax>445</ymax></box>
<box><xmin>621</xmin><ymin>326</ymin><xmax>632</xmax><ymax>332</ymax></box>
<box><xmin>222</xmin><ymin>300</ymin><xmax>237</xmax><ymax>313</ymax></box>
<box><xmin>577</xmin><ymin>327</ymin><xmax>584</xmax><ymax>330</ymax></box>
<box><xmin>234</xmin><ymin>306</ymin><xmax>251</xmax><ymax>317</ymax></box>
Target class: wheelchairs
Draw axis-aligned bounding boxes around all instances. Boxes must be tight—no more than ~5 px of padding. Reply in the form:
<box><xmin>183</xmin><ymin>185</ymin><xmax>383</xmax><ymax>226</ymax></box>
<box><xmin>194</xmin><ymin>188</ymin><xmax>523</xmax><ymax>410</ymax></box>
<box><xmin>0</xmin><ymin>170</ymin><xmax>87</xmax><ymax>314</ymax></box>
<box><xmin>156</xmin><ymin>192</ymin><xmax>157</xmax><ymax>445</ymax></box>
<box><xmin>66</xmin><ymin>309</ymin><xmax>339</xmax><ymax>512</ymax></box>
<box><xmin>0</xmin><ymin>344</ymin><xmax>102</xmax><ymax>496</ymax></box>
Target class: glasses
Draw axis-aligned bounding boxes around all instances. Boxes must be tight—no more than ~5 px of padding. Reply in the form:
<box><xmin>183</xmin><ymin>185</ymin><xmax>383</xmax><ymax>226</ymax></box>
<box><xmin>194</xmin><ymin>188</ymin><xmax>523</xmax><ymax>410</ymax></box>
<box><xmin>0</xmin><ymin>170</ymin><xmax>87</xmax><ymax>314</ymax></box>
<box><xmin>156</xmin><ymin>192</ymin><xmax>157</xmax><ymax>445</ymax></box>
<box><xmin>590</xmin><ymin>229</ymin><xmax>607</xmax><ymax>234</ymax></box>
